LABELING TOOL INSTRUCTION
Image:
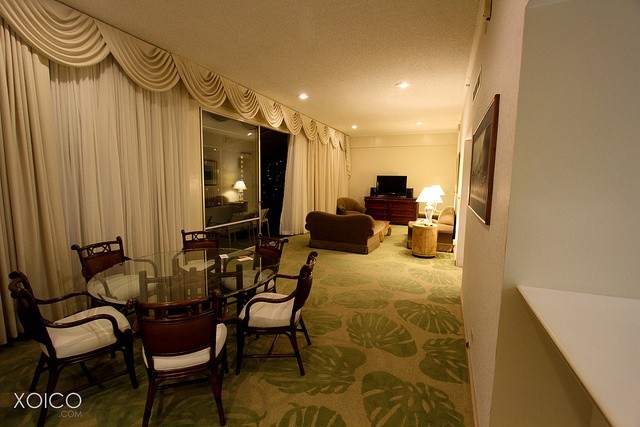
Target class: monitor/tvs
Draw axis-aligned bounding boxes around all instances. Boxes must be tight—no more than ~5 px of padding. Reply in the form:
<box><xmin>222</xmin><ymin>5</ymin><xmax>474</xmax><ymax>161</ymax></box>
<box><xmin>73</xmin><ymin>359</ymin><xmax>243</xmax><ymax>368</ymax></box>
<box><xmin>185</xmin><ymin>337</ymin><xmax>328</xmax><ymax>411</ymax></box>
<box><xmin>376</xmin><ymin>175</ymin><xmax>407</xmax><ymax>198</ymax></box>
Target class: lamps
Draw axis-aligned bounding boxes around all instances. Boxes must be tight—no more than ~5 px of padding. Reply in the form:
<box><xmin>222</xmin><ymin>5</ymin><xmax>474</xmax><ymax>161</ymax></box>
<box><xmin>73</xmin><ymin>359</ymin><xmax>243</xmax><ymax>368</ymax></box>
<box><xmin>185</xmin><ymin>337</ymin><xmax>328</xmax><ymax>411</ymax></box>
<box><xmin>415</xmin><ymin>187</ymin><xmax>442</xmax><ymax>226</ymax></box>
<box><xmin>432</xmin><ymin>185</ymin><xmax>445</xmax><ymax>208</ymax></box>
<box><xmin>233</xmin><ymin>180</ymin><xmax>247</xmax><ymax>201</ymax></box>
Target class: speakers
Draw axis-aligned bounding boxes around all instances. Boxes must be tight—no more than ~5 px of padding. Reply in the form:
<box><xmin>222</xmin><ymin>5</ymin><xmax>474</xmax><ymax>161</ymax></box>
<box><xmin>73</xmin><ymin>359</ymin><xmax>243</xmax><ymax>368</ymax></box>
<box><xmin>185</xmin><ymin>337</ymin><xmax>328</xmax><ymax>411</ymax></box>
<box><xmin>406</xmin><ymin>188</ymin><xmax>413</xmax><ymax>198</ymax></box>
<box><xmin>370</xmin><ymin>187</ymin><xmax>376</xmax><ymax>197</ymax></box>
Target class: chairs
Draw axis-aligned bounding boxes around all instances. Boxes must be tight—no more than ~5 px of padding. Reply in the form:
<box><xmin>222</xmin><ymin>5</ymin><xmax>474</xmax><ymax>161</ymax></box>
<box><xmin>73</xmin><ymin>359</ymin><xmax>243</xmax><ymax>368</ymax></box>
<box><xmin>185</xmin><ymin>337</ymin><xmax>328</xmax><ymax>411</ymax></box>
<box><xmin>8</xmin><ymin>271</ymin><xmax>139</xmax><ymax>426</ymax></box>
<box><xmin>245</xmin><ymin>208</ymin><xmax>271</xmax><ymax>243</ymax></box>
<box><xmin>126</xmin><ymin>288</ymin><xmax>230</xmax><ymax>427</ymax></box>
<box><xmin>222</xmin><ymin>234</ymin><xmax>289</xmax><ymax>338</ymax></box>
<box><xmin>207</xmin><ymin>214</ymin><xmax>232</xmax><ymax>248</ymax></box>
<box><xmin>71</xmin><ymin>236</ymin><xmax>149</xmax><ymax>338</ymax></box>
<box><xmin>180</xmin><ymin>228</ymin><xmax>219</xmax><ymax>248</ymax></box>
<box><xmin>235</xmin><ymin>250</ymin><xmax>318</xmax><ymax>375</ymax></box>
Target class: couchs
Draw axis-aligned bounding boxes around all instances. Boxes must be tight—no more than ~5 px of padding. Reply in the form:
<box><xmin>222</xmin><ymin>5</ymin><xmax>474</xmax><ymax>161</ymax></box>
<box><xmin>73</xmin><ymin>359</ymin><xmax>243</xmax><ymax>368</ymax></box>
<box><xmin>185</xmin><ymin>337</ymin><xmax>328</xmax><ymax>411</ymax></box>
<box><xmin>336</xmin><ymin>198</ymin><xmax>366</xmax><ymax>214</ymax></box>
<box><xmin>407</xmin><ymin>207</ymin><xmax>454</xmax><ymax>251</ymax></box>
<box><xmin>305</xmin><ymin>210</ymin><xmax>380</xmax><ymax>254</ymax></box>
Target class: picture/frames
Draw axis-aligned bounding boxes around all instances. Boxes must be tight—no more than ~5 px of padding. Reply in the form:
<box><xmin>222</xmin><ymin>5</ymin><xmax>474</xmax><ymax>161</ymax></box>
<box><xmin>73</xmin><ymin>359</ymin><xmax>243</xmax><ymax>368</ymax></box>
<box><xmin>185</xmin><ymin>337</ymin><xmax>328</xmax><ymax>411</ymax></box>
<box><xmin>204</xmin><ymin>161</ymin><xmax>217</xmax><ymax>186</ymax></box>
<box><xmin>467</xmin><ymin>93</ymin><xmax>500</xmax><ymax>224</ymax></box>
<box><xmin>454</xmin><ymin>152</ymin><xmax>460</xmax><ymax>193</ymax></box>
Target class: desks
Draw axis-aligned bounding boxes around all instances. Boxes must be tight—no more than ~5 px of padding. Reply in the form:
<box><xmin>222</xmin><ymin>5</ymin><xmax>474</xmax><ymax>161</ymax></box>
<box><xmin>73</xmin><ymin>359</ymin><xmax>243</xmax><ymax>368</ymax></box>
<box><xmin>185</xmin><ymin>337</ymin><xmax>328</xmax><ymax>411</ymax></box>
<box><xmin>364</xmin><ymin>197</ymin><xmax>419</xmax><ymax>224</ymax></box>
<box><xmin>230</xmin><ymin>201</ymin><xmax>248</xmax><ymax>213</ymax></box>
<box><xmin>88</xmin><ymin>249</ymin><xmax>280</xmax><ymax>316</ymax></box>
